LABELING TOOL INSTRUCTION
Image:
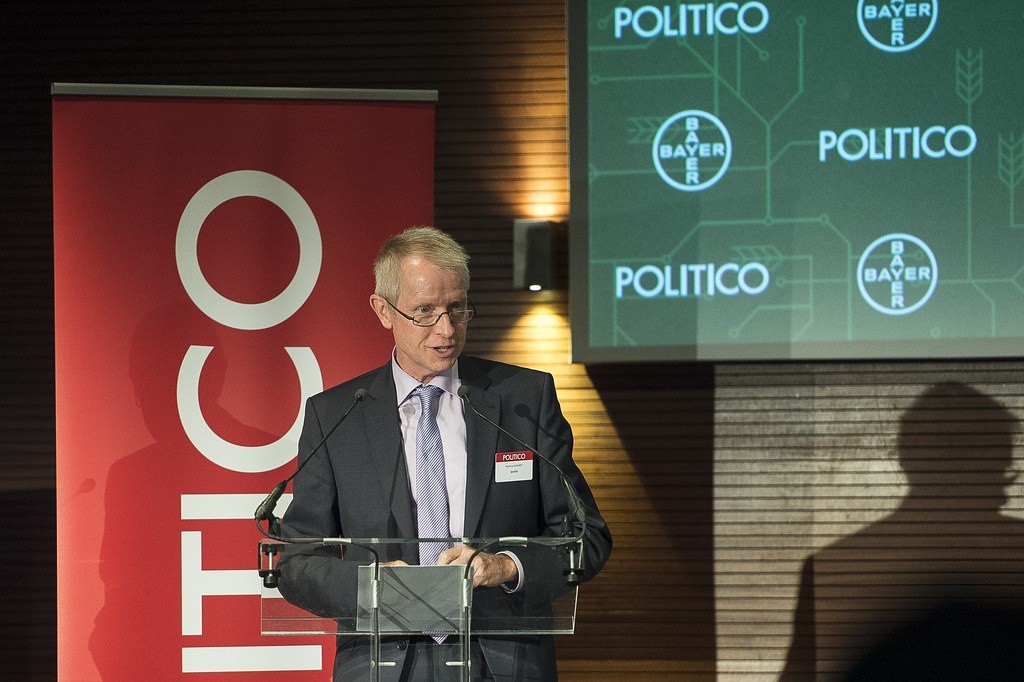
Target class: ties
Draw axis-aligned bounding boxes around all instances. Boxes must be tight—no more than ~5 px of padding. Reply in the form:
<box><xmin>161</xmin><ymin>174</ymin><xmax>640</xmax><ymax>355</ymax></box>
<box><xmin>410</xmin><ymin>384</ymin><xmax>450</xmax><ymax>644</ymax></box>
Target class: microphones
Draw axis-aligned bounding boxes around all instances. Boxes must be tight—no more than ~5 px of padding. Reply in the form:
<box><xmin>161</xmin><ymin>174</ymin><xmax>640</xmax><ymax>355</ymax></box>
<box><xmin>254</xmin><ymin>388</ymin><xmax>369</xmax><ymax>520</ymax></box>
<box><xmin>457</xmin><ymin>385</ymin><xmax>587</xmax><ymax>524</ymax></box>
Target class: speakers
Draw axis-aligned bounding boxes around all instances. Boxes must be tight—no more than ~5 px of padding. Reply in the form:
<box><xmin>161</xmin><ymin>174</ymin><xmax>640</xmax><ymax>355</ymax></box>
<box><xmin>511</xmin><ymin>220</ymin><xmax>570</xmax><ymax>291</ymax></box>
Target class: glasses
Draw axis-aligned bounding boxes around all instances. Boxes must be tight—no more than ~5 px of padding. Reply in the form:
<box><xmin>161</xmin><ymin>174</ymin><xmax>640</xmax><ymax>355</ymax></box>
<box><xmin>379</xmin><ymin>297</ymin><xmax>476</xmax><ymax>328</ymax></box>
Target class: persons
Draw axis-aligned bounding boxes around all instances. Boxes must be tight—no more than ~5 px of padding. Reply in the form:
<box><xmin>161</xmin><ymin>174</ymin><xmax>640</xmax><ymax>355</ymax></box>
<box><xmin>273</xmin><ymin>227</ymin><xmax>613</xmax><ymax>682</ymax></box>
